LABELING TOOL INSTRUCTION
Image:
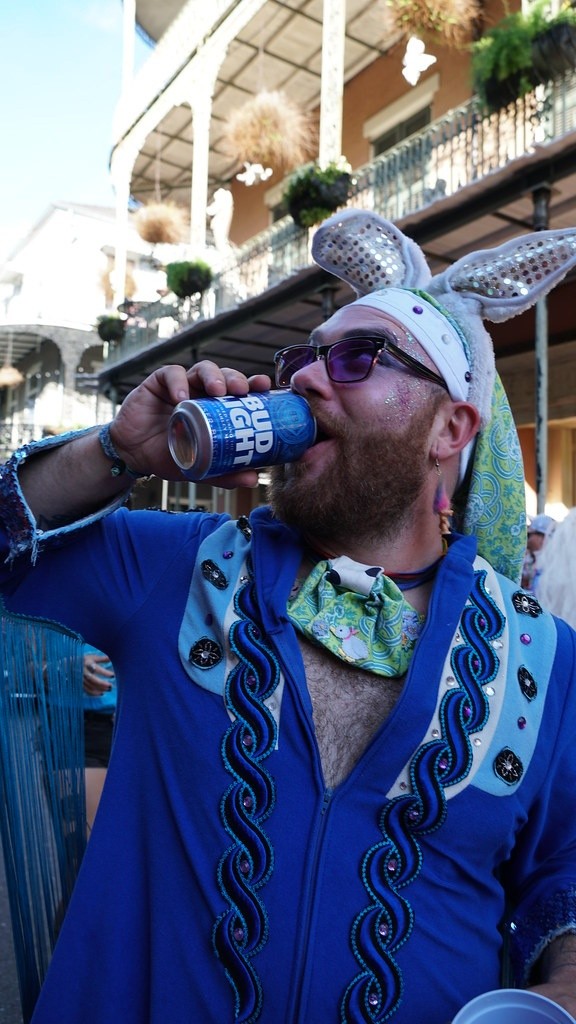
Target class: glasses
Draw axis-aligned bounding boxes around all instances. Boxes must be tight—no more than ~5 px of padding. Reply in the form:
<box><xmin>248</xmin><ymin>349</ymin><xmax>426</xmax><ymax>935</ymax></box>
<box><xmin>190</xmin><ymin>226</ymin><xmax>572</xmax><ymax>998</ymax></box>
<box><xmin>274</xmin><ymin>334</ymin><xmax>450</xmax><ymax>393</ymax></box>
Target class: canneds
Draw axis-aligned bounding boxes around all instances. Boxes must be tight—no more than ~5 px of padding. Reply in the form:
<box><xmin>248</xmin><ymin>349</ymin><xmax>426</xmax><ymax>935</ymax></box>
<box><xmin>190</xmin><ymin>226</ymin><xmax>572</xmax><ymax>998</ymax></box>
<box><xmin>167</xmin><ymin>389</ymin><xmax>318</xmax><ymax>481</ymax></box>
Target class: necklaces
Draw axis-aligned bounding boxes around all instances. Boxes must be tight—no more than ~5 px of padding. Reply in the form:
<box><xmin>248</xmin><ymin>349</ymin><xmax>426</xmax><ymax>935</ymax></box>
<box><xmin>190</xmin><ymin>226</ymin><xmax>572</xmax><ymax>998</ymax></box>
<box><xmin>298</xmin><ymin>522</ymin><xmax>448</xmax><ymax>682</ymax></box>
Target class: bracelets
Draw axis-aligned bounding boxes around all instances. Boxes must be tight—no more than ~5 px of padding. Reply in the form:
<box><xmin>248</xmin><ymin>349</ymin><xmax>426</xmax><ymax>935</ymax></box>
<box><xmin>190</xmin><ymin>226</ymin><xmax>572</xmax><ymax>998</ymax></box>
<box><xmin>97</xmin><ymin>420</ymin><xmax>148</xmax><ymax>483</ymax></box>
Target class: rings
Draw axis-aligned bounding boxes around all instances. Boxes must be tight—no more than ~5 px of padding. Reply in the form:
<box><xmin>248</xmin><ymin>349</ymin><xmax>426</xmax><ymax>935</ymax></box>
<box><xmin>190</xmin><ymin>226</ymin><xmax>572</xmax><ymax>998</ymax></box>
<box><xmin>86</xmin><ymin>663</ymin><xmax>97</xmax><ymax>672</ymax></box>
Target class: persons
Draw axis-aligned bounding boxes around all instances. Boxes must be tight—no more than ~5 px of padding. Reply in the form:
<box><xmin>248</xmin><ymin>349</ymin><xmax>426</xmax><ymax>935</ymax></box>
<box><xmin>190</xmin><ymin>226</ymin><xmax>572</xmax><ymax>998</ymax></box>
<box><xmin>1</xmin><ymin>609</ymin><xmax>119</xmax><ymax>850</ymax></box>
<box><xmin>517</xmin><ymin>517</ymin><xmax>559</xmax><ymax>594</ymax></box>
<box><xmin>0</xmin><ymin>292</ymin><xmax>576</xmax><ymax>1024</ymax></box>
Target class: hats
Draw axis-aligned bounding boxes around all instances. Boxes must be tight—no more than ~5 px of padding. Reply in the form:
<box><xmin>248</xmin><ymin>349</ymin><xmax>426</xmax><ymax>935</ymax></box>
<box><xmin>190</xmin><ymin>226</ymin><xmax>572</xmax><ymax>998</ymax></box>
<box><xmin>531</xmin><ymin>515</ymin><xmax>557</xmax><ymax>537</ymax></box>
